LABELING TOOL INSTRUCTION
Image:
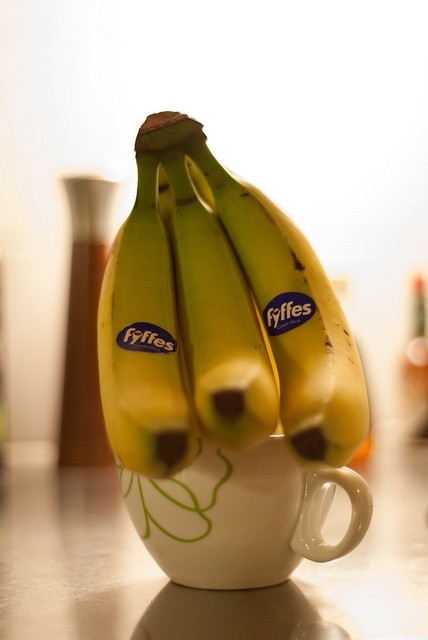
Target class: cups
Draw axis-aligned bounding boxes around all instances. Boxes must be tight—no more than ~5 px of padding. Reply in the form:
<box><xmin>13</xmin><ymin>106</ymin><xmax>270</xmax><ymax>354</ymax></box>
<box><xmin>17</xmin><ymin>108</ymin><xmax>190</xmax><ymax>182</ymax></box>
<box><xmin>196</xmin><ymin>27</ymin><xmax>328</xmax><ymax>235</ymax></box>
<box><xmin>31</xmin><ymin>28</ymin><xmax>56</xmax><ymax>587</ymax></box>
<box><xmin>109</xmin><ymin>425</ymin><xmax>375</xmax><ymax>592</ymax></box>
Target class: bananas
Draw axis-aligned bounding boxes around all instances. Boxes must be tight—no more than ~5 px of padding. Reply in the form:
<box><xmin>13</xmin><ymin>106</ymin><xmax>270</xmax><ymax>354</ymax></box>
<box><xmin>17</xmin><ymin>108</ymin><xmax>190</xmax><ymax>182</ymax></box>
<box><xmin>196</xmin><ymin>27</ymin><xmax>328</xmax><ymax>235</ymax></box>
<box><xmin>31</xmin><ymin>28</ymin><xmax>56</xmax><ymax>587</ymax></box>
<box><xmin>100</xmin><ymin>111</ymin><xmax>371</xmax><ymax>480</ymax></box>
